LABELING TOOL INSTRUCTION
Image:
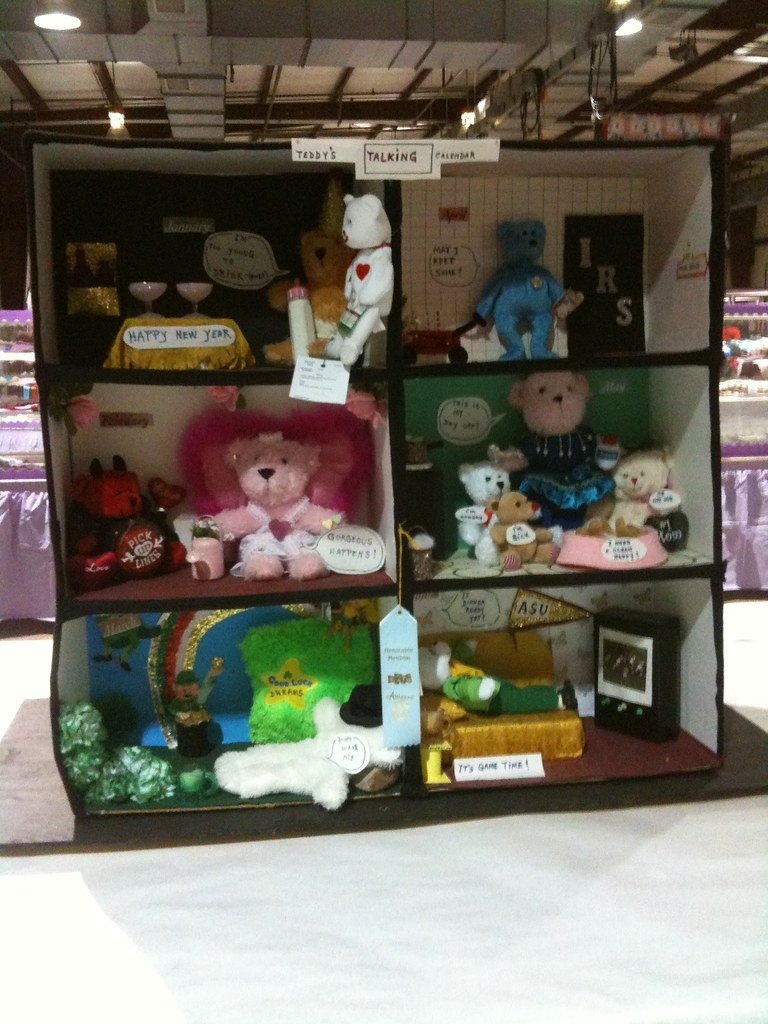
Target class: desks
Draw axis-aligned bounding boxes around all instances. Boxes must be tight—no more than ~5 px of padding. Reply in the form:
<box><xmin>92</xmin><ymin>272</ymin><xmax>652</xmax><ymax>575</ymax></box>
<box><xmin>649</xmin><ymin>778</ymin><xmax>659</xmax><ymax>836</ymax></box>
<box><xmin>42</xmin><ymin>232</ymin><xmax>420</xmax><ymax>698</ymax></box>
<box><xmin>105</xmin><ymin>318</ymin><xmax>252</xmax><ymax>371</ymax></box>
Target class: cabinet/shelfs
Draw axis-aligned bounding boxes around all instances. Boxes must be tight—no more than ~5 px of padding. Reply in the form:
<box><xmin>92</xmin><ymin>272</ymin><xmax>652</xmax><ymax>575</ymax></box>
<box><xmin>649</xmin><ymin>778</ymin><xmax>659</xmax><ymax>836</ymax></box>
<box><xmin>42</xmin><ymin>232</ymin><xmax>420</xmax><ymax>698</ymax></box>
<box><xmin>25</xmin><ymin>130</ymin><xmax>731</xmax><ymax>842</ymax></box>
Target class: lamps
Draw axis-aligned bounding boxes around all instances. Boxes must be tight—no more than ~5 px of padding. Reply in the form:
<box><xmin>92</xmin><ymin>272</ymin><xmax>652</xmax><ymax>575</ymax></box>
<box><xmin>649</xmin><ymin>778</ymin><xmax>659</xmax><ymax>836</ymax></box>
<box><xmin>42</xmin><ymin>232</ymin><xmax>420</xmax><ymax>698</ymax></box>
<box><xmin>34</xmin><ymin>0</ymin><xmax>81</xmax><ymax>31</ymax></box>
<box><xmin>108</xmin><ymin>61</ymin><xmax>125</xmax><ymax>129</ymax></box>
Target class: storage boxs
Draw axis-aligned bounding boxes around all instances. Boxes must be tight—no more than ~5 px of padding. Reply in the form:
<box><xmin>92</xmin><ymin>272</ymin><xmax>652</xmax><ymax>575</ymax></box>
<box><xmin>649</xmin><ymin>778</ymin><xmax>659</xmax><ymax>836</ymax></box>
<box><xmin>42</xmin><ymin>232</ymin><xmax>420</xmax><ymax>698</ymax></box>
<box><xmin>595</xmin><ymin>607</ymin><xmax>680</xmax><ymax>740</ymax></box>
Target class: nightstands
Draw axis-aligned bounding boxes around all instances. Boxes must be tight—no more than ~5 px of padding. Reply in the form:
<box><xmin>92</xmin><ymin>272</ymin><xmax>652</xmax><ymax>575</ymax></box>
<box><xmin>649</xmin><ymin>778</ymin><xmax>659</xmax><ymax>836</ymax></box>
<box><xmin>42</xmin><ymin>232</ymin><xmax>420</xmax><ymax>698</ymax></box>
<box><xmin>420</xmin><ymin>740</ymin><xmax>451</xmax><ymax>783</ymax></box>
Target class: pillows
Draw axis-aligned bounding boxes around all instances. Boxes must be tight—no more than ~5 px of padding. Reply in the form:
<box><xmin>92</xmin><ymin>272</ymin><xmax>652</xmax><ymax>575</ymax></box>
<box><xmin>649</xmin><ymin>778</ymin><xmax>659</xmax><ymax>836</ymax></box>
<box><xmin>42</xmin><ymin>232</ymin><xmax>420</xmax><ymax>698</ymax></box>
<box><xmin>237</xmin><ymin>618</ymin><xmax>374</xmax><ymax>745</ymax></box>
<box><xmin>421</xmin><ymin>695</ymin><xmax>467</xmax><ymax>722</ymax></box>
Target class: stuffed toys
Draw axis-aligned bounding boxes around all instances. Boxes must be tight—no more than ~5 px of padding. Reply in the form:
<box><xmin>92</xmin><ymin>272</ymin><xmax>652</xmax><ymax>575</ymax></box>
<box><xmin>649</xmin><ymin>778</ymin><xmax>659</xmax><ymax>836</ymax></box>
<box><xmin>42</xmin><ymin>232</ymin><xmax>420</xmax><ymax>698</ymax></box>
<box><xmin>211</xmin><ymin>435</ymin><xmax>345</xmax><ymax>578</ymax></box>
<box><xmin>68</xmin><ymin>178</ymin><xmax>679</xmax><ymax>812</ymax></box>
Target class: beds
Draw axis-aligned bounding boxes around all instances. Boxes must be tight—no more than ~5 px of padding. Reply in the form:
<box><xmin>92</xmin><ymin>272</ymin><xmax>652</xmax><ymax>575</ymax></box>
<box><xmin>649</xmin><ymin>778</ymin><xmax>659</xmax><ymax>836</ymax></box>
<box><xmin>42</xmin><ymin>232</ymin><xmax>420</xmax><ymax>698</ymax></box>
<box><xmin>416</xmin><ymin>709</ymin><xmax>585</xmax><ymax>764</ymax></box>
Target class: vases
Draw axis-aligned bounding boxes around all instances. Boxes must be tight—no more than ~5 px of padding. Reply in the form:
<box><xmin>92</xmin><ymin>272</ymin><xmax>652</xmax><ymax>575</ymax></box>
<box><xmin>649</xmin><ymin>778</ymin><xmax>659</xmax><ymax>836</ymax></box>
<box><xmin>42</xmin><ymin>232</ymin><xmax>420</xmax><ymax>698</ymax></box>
<box><xmin>419</xmin><ymin>705</ymin><xmax>451</xmax><ymax>743</ymax></box>
